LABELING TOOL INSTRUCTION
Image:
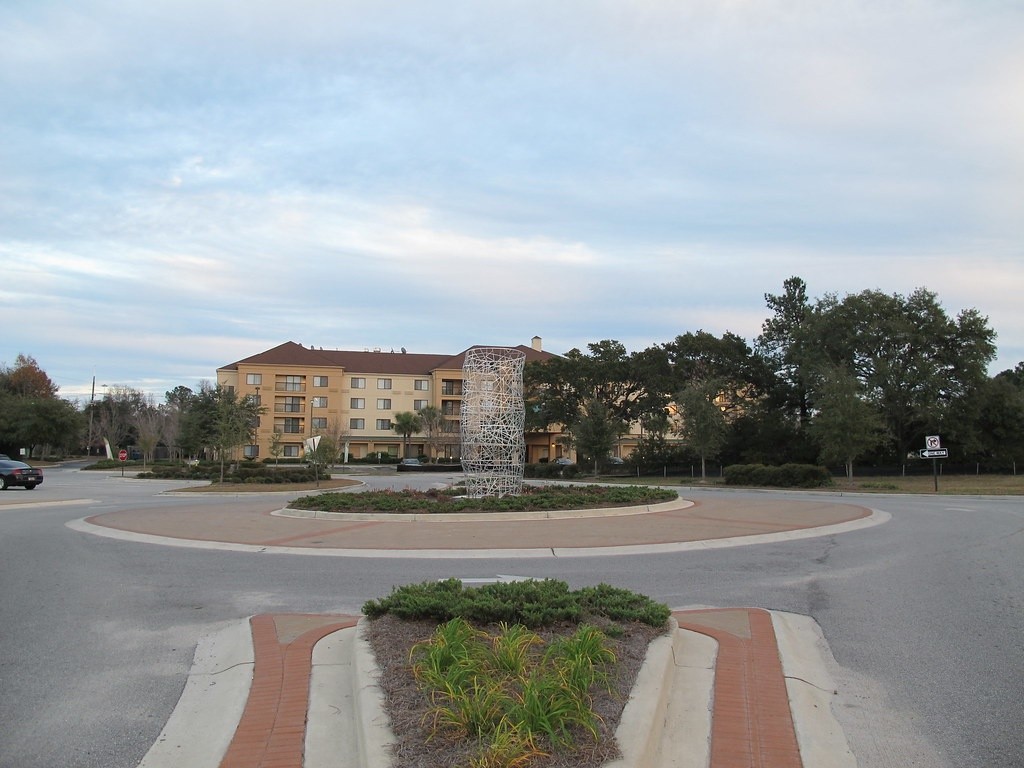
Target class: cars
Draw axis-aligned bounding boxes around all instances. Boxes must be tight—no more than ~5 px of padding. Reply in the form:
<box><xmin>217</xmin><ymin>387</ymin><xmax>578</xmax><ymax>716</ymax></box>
<box><xmin>549</xmin><ymin>458</ymin><xmax>574</xmax><ymax>470</ymax></box>
<box><xmin>396</xmin><ymin>458</ymin><xmax>423</xmax><ymax>471</ymax></box>
<box><xmin>0</xmin><ymin>453</ymin><xmax>43</xmax><ymax>490</ymax></box>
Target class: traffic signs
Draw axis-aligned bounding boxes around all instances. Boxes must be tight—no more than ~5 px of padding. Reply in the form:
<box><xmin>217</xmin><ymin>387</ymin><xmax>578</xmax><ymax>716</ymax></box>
<box><xmin>919</xmin><ymin>448</ymin><xmax>948</xmax><ymax>459</ymax></box>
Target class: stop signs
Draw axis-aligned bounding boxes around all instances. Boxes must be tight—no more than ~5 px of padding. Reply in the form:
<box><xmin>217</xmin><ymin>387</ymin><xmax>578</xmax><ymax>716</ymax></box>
<box><xmin>119</xmin><ymin>450</ymin><xmax>128</xmax><ymax>461</ymax></box>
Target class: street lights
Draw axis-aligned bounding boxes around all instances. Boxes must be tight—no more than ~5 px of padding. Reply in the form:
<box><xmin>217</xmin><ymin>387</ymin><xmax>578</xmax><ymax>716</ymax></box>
<box><xmin>254</xmin><ymin>385</ymin><xmax>260</xmax><ymax>462</ymax></box>
<box><xmin>310</xmin><ymin>399</ymin><xmax>314</xmax><ymax>438</ymax></box>
